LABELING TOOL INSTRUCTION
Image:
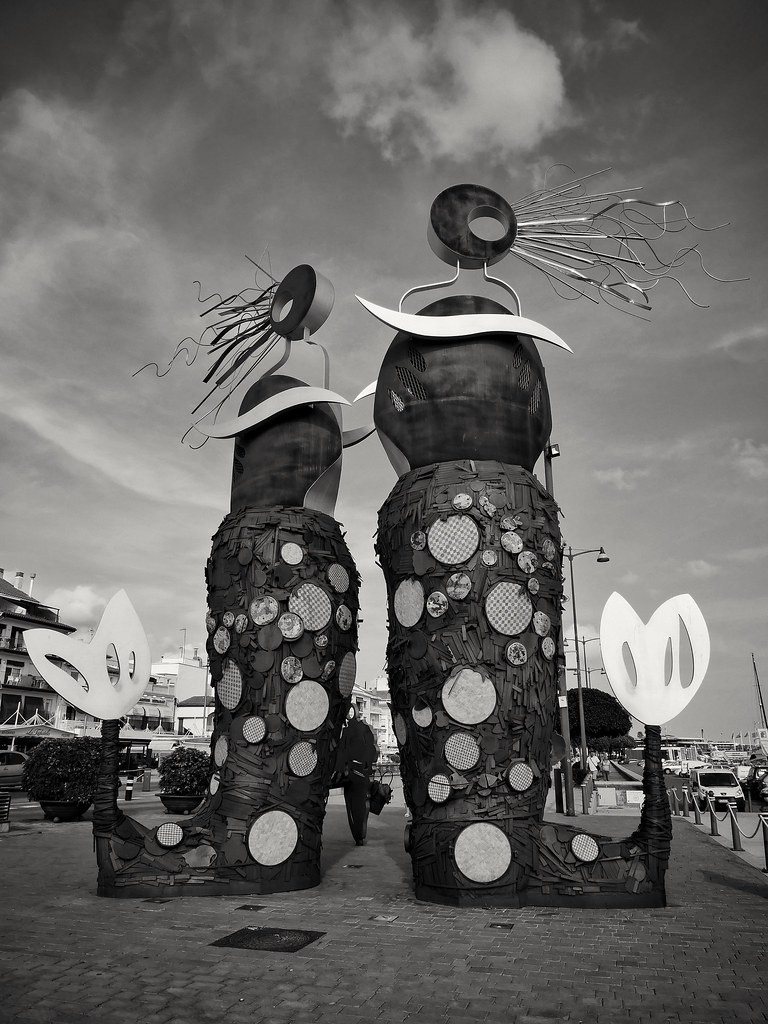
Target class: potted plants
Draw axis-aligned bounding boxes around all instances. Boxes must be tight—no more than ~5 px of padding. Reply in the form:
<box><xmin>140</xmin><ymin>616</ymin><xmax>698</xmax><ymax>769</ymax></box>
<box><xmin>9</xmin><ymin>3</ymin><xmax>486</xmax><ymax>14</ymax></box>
<box><xmin>155</xmin><ymin>747</ymin><xmax>212</xmax><ymax>813</ymax></box>
<box><xmin>21</xmin><ymin>737</ymin><xmax>103</xmax><ymax>820</ymax></box>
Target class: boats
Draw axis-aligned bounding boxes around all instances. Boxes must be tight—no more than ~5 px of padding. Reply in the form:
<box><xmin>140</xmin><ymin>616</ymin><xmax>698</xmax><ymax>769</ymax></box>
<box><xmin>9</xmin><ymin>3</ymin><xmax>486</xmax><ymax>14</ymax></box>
<box><xmin>706</xmin><ymin>728</ymin><xmax>768</xmax><ymax>771</ymax></box>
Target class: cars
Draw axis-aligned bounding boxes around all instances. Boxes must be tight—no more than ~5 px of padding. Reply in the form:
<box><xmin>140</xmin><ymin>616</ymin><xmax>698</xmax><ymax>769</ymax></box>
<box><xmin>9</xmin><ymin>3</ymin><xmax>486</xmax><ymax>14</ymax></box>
<box><xmin>0</xmin><ymin>750</ymin><xmax>33</xmax><ymax>791</ymax></box>
<box><xmin>632</xmin><ymin>758</ymin><xmax>768</xmax><ymax>804</ymax></box>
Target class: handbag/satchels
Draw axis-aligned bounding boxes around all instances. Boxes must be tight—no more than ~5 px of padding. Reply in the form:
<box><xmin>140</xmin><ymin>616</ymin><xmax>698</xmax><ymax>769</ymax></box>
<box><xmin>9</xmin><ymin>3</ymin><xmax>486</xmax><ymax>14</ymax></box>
<box><xmin>370</xmin><ymin>767</ymin><xmax>394</xmax><ymax>804</ymax></box>
<box><xmin>343</xmin><ymin>758</ymin><xmax>369</xmax><ymax>785</ymax></box>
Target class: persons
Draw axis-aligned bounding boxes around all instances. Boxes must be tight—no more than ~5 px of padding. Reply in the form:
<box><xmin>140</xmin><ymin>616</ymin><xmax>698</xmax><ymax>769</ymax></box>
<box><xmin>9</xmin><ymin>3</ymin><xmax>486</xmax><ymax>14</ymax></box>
<box><xmin>585</xmin><ymin>750</ymin><xmax>613</xmax><ymax>781</ymax></box>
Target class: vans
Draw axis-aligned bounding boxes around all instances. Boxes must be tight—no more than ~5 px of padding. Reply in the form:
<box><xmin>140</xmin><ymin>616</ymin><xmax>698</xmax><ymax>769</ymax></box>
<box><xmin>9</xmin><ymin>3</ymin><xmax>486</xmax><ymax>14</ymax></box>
<box><xmin>688</xmin><ymin>769</ymin><xmax>747</xmax><ymax>812</ymax></box>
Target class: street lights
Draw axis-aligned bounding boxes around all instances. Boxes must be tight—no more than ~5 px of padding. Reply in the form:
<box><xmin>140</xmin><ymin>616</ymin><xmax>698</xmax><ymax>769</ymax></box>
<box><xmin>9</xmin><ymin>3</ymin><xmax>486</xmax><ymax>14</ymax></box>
<box><xmin>561</xmin><ymin>546</ymin><xmax>611</xmax><ymax>818</ymax></box>
